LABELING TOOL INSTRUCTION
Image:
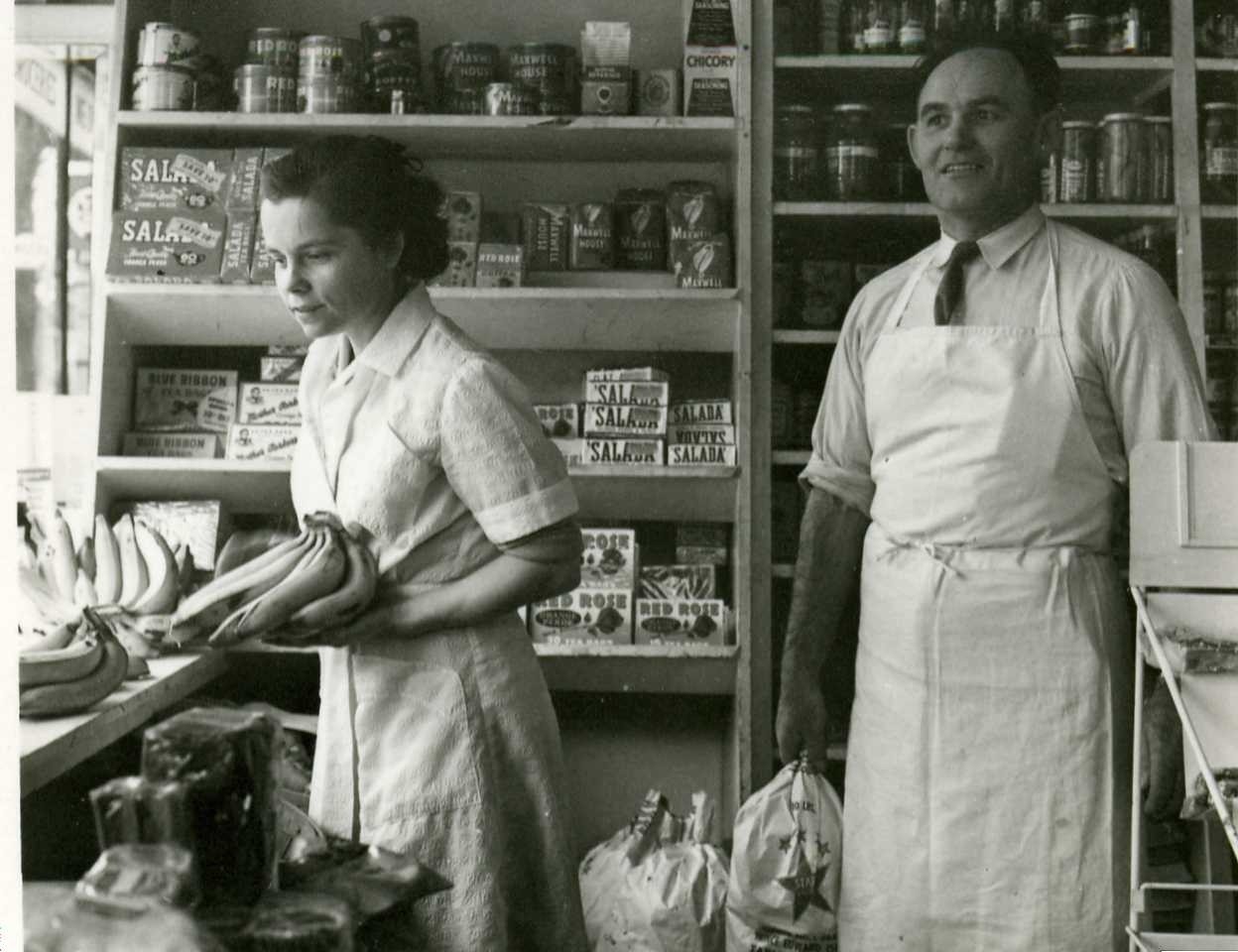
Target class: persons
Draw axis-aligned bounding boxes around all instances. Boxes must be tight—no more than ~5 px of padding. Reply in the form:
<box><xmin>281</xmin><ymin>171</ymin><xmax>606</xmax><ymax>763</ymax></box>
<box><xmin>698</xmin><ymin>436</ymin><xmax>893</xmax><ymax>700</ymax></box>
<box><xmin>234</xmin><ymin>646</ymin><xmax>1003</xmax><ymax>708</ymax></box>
<box><xmin>773</xmin><ymin>29</ymin><xmax>1227</xmax><ymax>952</ymax></box>
<box><xmin>257</xmin><ymin>133</ymin><xmax>586</xmax><ymax>952</ymax></box>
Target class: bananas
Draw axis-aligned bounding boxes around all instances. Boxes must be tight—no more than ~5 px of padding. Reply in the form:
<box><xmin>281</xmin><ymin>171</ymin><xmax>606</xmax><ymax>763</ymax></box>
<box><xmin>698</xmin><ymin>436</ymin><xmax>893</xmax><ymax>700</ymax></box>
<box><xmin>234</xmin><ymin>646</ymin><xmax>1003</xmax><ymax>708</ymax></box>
<box><xmin>168</xmin><ymin>511</ymin><xmax>376</xmax><ymax>648</ymax></box>
<box><xmin>18</xmin><ymin>506</ymin><xmax>199</xmax><ymax>721</ymax></box>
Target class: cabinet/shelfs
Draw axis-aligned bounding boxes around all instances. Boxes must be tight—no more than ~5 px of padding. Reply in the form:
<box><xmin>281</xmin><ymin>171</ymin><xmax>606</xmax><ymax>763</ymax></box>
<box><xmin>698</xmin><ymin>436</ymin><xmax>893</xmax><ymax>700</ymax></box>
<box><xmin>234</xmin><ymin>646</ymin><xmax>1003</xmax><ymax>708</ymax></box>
<box><xmin>757</xmin><ymin>0</ymin><xmax>1238</xmax><ymax>798</ymax></box>
<box><xmin>82</xmin><ymin>0</ymin><xmax>754</xmax><ymax>838</ymax></box>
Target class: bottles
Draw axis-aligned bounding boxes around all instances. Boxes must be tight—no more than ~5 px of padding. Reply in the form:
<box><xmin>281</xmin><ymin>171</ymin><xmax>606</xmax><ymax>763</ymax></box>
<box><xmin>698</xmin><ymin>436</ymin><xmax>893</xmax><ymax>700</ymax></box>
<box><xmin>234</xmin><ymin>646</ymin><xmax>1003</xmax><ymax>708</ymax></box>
<box><xmin>774</xmin><ymin>0</ymin><xmax>1238</xmax><ymax>450</ymax></box>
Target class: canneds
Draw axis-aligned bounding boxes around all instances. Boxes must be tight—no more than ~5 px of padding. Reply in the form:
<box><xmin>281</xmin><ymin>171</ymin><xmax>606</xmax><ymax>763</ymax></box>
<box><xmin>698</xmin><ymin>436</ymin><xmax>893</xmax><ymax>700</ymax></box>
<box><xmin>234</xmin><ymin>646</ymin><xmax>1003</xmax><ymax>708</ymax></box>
<box><xmin>130</xmin><ymin>15</ymin><xmax>581</xmax><ymax>118</ymax></box>
<box><xmin>1199</xmin><ymin>268</ymin><xmax>1238</xmax><ymax>339</ymax></box>
<box><xmin>773</xmin><ymin>99</ymin><xmax>1238</xmax><ymax>206</ymax></box>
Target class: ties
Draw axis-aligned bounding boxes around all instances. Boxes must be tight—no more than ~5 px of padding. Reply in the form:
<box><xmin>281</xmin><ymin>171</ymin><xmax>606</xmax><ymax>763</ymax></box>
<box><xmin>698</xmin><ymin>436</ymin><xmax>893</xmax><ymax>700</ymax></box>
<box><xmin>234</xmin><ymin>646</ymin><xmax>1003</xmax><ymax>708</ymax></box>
<box><xmin>933</xmin><ymin>242</ymin><xmax>980</xmax><ymax>325</ymax></box>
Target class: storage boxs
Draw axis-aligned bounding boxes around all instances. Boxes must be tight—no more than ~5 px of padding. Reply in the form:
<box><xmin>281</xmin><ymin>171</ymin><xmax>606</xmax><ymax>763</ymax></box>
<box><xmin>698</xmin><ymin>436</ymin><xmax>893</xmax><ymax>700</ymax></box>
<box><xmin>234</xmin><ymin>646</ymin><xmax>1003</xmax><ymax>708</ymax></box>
<box><xmin>104</xmin><ymin>149</ymin><xmax>738</xmax><ymax>647</ymax></box>
